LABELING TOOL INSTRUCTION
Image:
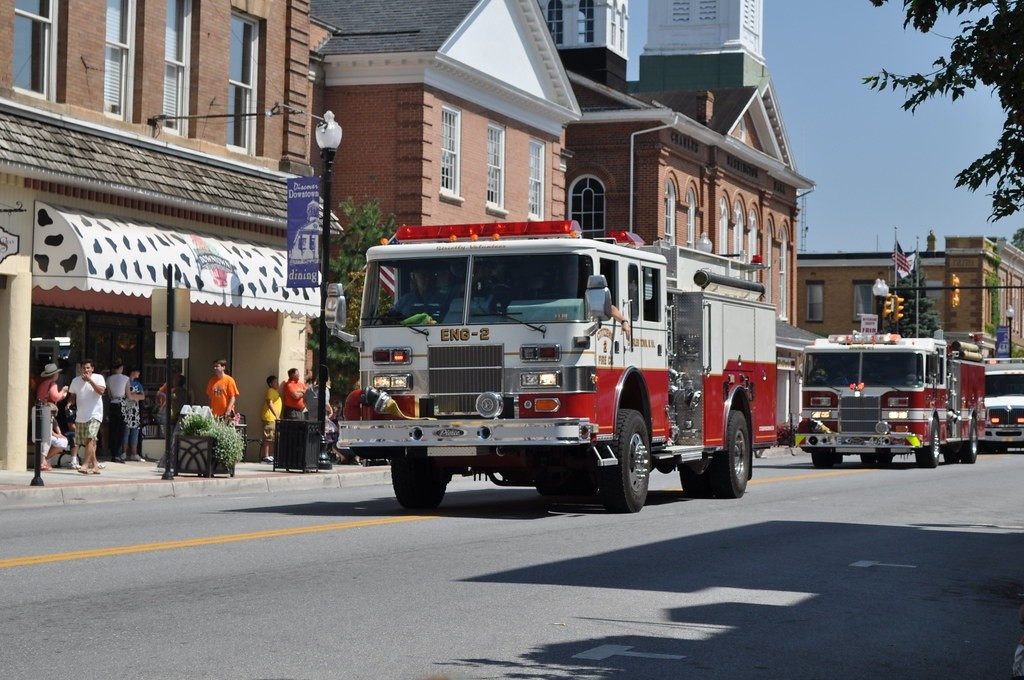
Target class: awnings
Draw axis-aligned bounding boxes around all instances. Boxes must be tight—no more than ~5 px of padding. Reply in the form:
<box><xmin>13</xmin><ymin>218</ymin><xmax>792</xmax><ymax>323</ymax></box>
<box><xmin>33</xmin><ymin>200</ymin><xmax>321</xmax><ymax>320</ymax></box>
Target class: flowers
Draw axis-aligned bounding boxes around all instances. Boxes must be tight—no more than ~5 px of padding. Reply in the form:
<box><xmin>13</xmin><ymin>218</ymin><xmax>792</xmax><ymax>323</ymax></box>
<box><xmin>776</xmin><ymin>422</ymin><xmax>799</xmax><ymax>441</ymax></box>
<box><xmin>180</xmin><ymin>415</ymin><xmax>248</xmax><ymax>469</ymax></box>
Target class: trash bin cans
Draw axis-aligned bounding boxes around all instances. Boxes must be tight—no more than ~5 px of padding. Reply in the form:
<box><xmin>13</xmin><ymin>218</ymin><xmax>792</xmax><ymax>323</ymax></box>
<box><xmin>274</xmin><ymin>419</ymin><xmax>322</xmax><ymax>473</ymax></box>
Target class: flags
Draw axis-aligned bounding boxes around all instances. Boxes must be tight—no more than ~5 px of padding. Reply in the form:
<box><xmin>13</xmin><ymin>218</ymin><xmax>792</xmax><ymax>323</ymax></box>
<box><xmin>894</xmin><ymin>251</ymin><xmax>916</xmax><ymax>279</ymax></box>
<box><xmin>891</xmin><ymin>241</ymin><xmax>910</xmax><ymax>273</ymax></box>
<box><xmin>365</xmin><ymin>235</ymin><xmax>399</xmax><ymax>295</ymax></box>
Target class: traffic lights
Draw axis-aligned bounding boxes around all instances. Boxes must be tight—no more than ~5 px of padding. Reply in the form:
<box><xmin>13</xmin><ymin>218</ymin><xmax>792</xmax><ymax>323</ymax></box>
<box><xmin>882</xmin><ymin>292</ymin><xmax>894</xmax><ymax>318</ymax></box>
<box><xmin>892</xmin><ymin>295</ymin><xmax>904</xmax><ymax>322</ymax></box>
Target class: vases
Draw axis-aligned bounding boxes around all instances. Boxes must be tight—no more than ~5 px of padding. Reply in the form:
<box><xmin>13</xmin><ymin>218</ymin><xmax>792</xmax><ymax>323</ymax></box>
<box><xmin>779</xmin><ymin>436</ymin><xmax>795</xmax><ymax>445</ymax></box>
<box><xmin>175</xmin><ymin>435</ymin><xmax>235</xmax><ymax>478</ymax></box>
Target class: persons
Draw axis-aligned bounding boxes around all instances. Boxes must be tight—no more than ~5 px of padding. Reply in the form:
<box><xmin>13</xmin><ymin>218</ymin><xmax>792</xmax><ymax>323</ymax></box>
<box><xmin>610</xmin><ymin>305</ymin><xmax>631</xmax><ymax>342</ymax></box>
<box><xmin>283</xmin><ymin>368</ymin><xmax>333</xmax><ymax>420</ymax></box>
<box><xmin>205</xmin><ymin>357</ymin><xmax>239</xmax><ymax>420</ymax></box>
<box><xmin>106</xmin><ymin>358</ymin><xmax>146</xmax><ymax>463</ymax></box>
<box><xmin>392</xmin><ymin>264</ymin><xmax>450</xmax><ymax>319</ymax></box>
<box><xmin>260</xmin><ymin>376</ymin><xmax>282</xmax><ymax>462</ymax></box>
<box><xmin>157</xmin><ymin>369</ymin><xmax>194</xmax><ymax>418</ymax></box>
<box><xmin>35</xmin><ymin>359</ymin><xmax>105</xmax><ymax>474</ymax></box>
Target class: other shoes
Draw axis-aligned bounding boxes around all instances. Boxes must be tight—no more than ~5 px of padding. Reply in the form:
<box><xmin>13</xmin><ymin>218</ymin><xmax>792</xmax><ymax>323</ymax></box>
<box><xmin>40</xmin><ymin>464</ymin><xmax>52</xmax><ymax>471</ymax></box>
<box><xmin>89</xmin><ymin>462</ymin><xmax>105</xmax><ymax>469</ymax></box>
<box><xmin>88</xmin><ymin>470</ymin><xmax>101</xmax><ymax>474</ymax></box>
<box><xmin>70</xmin><ymin>462</ymin><xmax>81</xmax><ymax>469</ymax></box>
<box><xmin>78</xmin><ymin>469</ymin><xmax>88</xmax><ymax>474</ymax></box>
<box><xmin>131</xmin><ymin>455</ymin><xmax>145</xmax><ymax>462</ymax></box>
<box><xmin>121</xmin><ymin>453</ymin><xmax>127</xmax><ymax>460</ymax></box>
<box><xmin>261</xmin><ymin>456</ymin><xmax>275</xmax><ymax>465</ymax></box>
<box><xmin>111</xmin><ymin>457</ymin><xmax>125</xmax><ymax>463</ymax></box>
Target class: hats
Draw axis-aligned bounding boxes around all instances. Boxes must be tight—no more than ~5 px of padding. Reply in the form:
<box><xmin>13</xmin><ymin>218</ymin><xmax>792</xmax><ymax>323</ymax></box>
<box><xmin>41</xmin><ymin>364</ymin><xmax>62</xmax><ymax>377</ymax></box>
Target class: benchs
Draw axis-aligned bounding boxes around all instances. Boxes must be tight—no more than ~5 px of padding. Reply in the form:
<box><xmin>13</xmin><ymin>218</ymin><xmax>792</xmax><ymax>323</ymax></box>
<box><xmin>55</xmin><ymin>435</ymin><xmax>85</xmax><ymax>468</ymax></box>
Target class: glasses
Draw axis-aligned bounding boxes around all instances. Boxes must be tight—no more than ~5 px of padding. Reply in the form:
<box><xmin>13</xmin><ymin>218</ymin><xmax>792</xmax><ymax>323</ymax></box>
<box><xmin>415</xmin><ymin>271</ymin><xmax>440</xmax><ymax>282</ymax></box>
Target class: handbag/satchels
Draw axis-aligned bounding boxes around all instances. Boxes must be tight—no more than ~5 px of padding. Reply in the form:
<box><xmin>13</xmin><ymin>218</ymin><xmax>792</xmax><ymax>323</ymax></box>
<box><xmin>48</xmin><ymin>403</ymin><xmax>57</xmax><ymax>416</ymax></box>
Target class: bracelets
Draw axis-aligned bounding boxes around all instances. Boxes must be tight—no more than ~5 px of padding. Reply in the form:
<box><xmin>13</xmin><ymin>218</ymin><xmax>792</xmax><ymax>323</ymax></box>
<box><xmin>621</xmin><ymin>320</ymin><xmax>628</xmax><ymax>324</ymax></box>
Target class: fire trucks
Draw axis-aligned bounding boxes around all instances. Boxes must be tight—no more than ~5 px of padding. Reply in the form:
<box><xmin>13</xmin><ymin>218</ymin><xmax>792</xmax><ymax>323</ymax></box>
<box><xmin>792</xmin><ymin>331</ymin><xmax>987</xmax><ymax>469</ymax></box>
<box><xmin>977</xmin><ymin>356</ymin><xmax>1024</xmax><ymax>454</ymax></box>
<box><xmin>324</xmin><ymin>218</ymin><xmax>783</xmax><ymax>515</ymax></box>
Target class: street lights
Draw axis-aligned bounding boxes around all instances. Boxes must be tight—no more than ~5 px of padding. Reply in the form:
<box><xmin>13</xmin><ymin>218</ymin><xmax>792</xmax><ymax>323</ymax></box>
<box><xmin>316</xmin><ymin>112</ymin><xmax>343</xmax><ymax>469</ymax></box>
<box><xmin>1004</xmin><ymin>303</ymin><xmax>1013</xmax><ymax>360</ymax></box>
<box><xmin>873</xmin><ymin>278</ymin><xmax>889</xmax><ymax>336</ymax></box>
